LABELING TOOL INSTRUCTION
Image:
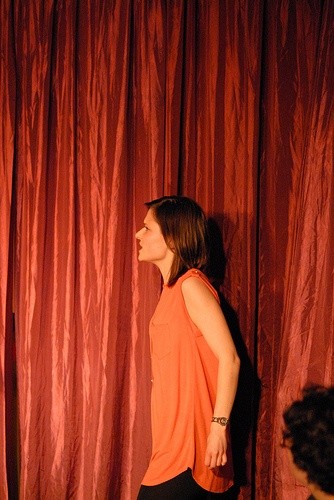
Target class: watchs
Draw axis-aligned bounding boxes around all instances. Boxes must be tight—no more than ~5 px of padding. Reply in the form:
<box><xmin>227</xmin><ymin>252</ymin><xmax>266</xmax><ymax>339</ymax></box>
<box><xmin>211</xmin><ymin>416</ymin><xmax>231</xmax><ymax>426</ymax></box>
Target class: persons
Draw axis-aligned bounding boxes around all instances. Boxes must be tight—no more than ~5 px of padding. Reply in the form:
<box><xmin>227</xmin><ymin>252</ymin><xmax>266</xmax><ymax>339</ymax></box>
<box><xmin>281</xmin><ymin>383</ymin><xmax>334</xmax><ymax>499</ymax></box>
<box><xmin>131</xmin><ymin>194</ymin><xmax>244</xmax><ymax>500</ymax></box>
